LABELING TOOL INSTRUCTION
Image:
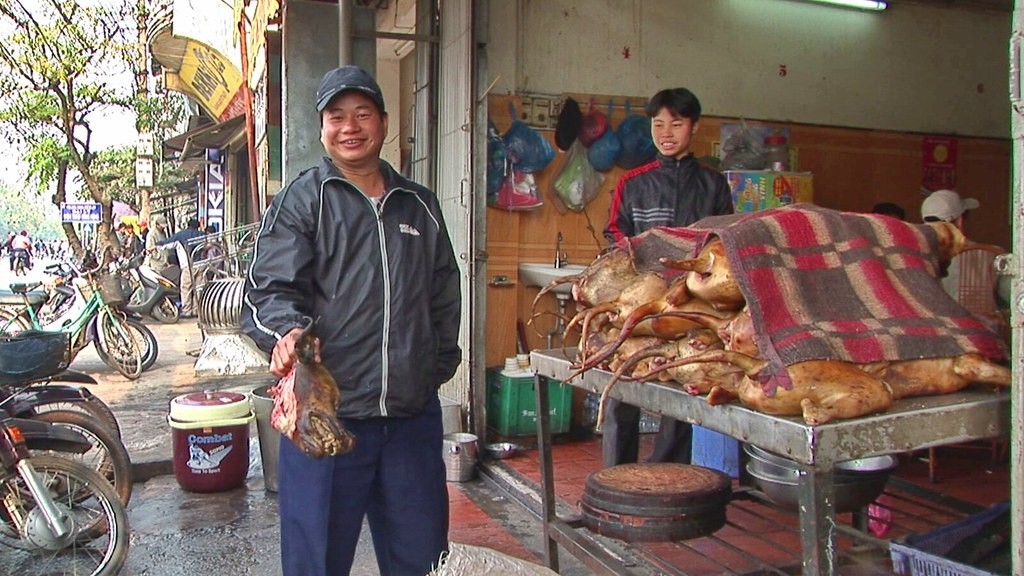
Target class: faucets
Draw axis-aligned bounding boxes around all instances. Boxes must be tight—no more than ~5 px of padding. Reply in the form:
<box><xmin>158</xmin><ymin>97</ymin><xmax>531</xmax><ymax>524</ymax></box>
<box><xmin>554</xmin><ymin>231</ymin><xmax>569</xmax><ymax>269</ymax></box>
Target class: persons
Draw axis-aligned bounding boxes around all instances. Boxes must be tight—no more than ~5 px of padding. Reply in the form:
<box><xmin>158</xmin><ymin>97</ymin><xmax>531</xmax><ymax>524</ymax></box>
<box><xmin>145</xmin><ymin>219</ymin><xmax>208</xmax><ymax>317</ymax></box>
<box><xmin>116</xmin><ymin>214</ymin><xmax>225</xmax><ymax>283</ymax></box>
<box><xmin>873</xmin><ymin>202</ymin><xmax>906</xmax><ymax>221</ymax></box>
<box><xmin>602</xmin><ymin>86</ymin><xmax>735</xmax><ymax>464</ymax></box>
<box><xmin>0</xmin><ymin>230</ymin><xmax>75</xmax><ymax>270</ymax></box>
<box><xmin>915</xmin><ymin>190</ymin><xmax>1012</xmax><ymax>462</ymax></box>
<box><xmin>239</xmin><ymin>66</ymin><xmax>462</xmax><ymax>576</ymax></box>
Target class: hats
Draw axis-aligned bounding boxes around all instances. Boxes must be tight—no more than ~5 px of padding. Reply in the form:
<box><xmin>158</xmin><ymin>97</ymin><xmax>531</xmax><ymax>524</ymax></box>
<box><xmin>315</xmin><ymin>65</ymin><xmax>385</xmax><ymax>113</ymax></box>
<box><xmin>922</xmin><ymin>190</ymin><xmax>981</xmax><ymax>225</ymax></box>
<box><xmin>556</xmin><ymin>96</ymin><xmax>584</xmax><ymax>151</ymax></box>
<box><xmin>156</xmin><ymin>215</ymin><xmax>170</xmax><ymax>228</ymax></box>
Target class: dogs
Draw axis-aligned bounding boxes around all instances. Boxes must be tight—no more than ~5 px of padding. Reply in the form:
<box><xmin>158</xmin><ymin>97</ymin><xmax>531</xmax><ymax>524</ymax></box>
<box><xmin>272</xmin><ymin>312</ymin><xmax>359</xmax><ymax>459</ymax></box>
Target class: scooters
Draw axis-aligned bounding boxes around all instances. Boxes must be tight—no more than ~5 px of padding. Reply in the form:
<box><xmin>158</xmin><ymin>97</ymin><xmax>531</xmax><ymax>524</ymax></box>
<box><xmin>0</xmin><ymin>218</ymin><xmax>185</xmax><ymax>576</ymax></box>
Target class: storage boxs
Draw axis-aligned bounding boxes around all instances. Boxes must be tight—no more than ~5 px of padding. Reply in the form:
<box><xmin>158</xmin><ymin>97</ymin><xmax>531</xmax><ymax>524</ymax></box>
<box><xmin>689</xmin><ymin>424</ymin><xmax>743</xmax><ymax>478</ymax></box>
<box><xmin>886</xmin><ymin>503</ymin><xmax>1011</xmax><ymax>576</ymax></box>
<box><xmin>486</xmin><ymin>366</ymin><xmax>573</xmax><ymax>438</ymax></box>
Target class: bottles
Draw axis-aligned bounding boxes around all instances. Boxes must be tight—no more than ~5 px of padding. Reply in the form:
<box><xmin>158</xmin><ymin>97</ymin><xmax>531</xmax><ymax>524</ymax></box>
<box><xmin>762</xmin><ymin>134</ymin><xmax>790</xmax><ymax>174</ymax></box>
<box><xmin>500</xmin><ymin>352</ymin><xmax>534</xmax><ymax>378</ymax></box>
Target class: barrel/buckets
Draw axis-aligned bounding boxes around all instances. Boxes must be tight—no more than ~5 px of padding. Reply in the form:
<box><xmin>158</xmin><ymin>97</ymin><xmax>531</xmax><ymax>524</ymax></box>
<box><xmin>166</xmin><ymin>389</ymin><xmax>257</xmax><ymax>494</ymax></box>
<box><xmin>442</xmin><ymin>432</ymin><xmax>481</xmax><ymax>482</ymax></box>
<box><xmin>251</xmin><ymin>385</ymin><xmax>282</xmax><ymax>492</ymax></box>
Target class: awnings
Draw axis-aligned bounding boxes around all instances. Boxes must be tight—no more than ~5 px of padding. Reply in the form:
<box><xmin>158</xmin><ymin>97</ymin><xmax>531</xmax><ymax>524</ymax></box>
<box><xmin>159</xmin><ymin>121</ymin><xmax>215</xmax><ymax>181</ymax></box>
<box><xmin>179</xmin><ymin>112</ymin><xmax>247</xmax><ymax>162</ymax></box>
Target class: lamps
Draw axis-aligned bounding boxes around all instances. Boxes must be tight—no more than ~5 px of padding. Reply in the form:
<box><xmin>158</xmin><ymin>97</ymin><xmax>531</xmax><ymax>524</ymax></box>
<box><xmin>823</xmin><ymin>0</ymin><xmax>887</xmax><ymax>11</ymax></box>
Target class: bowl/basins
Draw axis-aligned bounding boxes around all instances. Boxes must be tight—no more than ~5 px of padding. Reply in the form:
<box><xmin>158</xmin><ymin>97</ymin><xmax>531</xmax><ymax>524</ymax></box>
<box><xmin>743</xmin><ymin>438</ymin><xmax>896</xmax><ymax>513</ymax></box>
<box><xmin>486</xmin><ymin>442</ymin><xmax>519</xmax><ymax>459</ymax></box>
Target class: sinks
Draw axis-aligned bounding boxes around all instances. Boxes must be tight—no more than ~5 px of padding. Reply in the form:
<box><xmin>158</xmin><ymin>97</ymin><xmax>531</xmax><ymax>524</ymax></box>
<box><xmin>519</xmin><ymin>262</ymin><xmax>588</xmax><ymax>292</ymax></box>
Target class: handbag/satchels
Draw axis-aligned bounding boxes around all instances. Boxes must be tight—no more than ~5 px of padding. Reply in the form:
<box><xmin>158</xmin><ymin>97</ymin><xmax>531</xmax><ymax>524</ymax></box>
<box><xmin>502</xmin><ymin>102</ymin><xmax>557</xmax><ymax>174</ymax></box>
<box><xmin>494</xmin><ymin>167</ymin><xmax>543</xmax><ymax>211</ymax></box>
<box><xmin>550</xmin><ymin>138</ymin><xmax>605</xmax><ymax>216</ymax></box>
<box><xmin>488</xmin><ymin>118</ymin><xmax>508</xmax><ymax>197</ymax></box>
<box><xmin>588</xmin><ymin>100</ymin><xmax>622</xmax><ymax>172</ymax></box>
<box><xmin>580</xmin><ymin>98</ymin><xmax>608</xmax><ymax>146</ymax></box>
<box><xmin>616</xmin><ymin>101</ymin><xmax>658</xmax><ymax>170</ymax></box>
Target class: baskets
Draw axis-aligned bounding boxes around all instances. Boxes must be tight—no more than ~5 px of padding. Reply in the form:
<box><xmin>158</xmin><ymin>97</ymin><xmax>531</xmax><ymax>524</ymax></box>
<box><xmin>0</xmin><ymin>331</ymin><xmax>74</xmax><ymax>385</ymax></box>
<box><xmin>98</xmin><ymin>275</ymin><xmax>125</xmax><ymax>306</ymax></box>
